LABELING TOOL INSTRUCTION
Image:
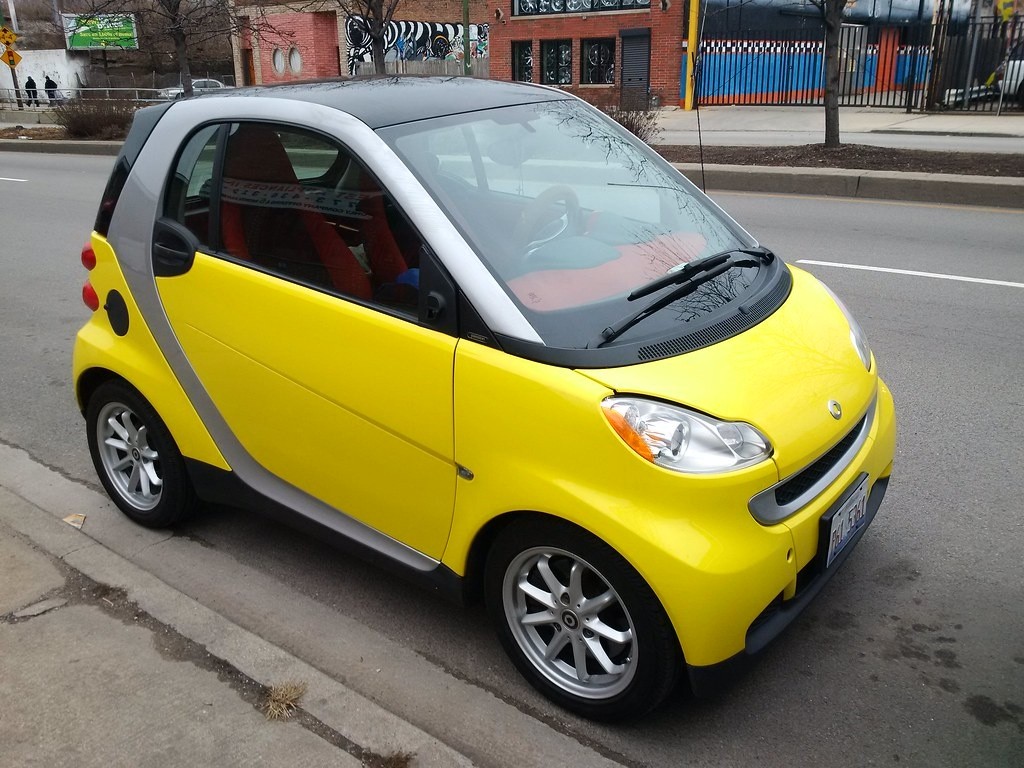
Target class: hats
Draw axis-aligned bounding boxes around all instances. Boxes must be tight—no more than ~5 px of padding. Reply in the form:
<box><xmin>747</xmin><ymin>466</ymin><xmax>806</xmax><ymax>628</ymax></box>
<box><xmin>45</xmin><ymin>76</ymin><xmax>49</xmax><ymax>78</ymax></box>
<box><xmin>28</xmin><ymin>76</ymin><xmax>32</xmax><ymax>78</ymax></box>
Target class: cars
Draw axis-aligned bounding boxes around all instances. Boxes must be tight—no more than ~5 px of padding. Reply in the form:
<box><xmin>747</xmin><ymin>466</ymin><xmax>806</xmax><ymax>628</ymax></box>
<box><xmin>157</xmin><ymin>78</ymin><xmax>235</xmax><ymax>100</ymax></box>
<box><xmin>63</xmin><ymin>75</ymin><xmax>893</xmax><ymax>714</ymax></box>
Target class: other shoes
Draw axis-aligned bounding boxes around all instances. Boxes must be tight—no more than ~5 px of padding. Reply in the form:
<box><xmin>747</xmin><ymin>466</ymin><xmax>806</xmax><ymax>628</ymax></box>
<box><xmin>36</xmin><ymin>103</ymin><xmax>40</xmax><ymax>107</ymax></box>
<box><xmin>26</xmin><ymin>103</ymin><xmax>31</xmax><ymax>107</ymax></box>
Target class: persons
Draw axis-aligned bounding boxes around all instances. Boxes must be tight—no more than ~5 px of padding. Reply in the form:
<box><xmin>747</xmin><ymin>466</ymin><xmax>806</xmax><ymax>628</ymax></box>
<box><xmin>25</xmin><ymin>76</ymin><xmax>40</xmax><ymax>107</ymax></box>
<box><xmin>45</xmin><ymin>76</ymin><xmax>57</xmax><ymax>107</ymax></box>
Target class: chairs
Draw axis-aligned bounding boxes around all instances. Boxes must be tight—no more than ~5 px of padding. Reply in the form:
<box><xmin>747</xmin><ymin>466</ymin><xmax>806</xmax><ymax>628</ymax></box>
<box><xmin>357</xmin><ymin>152</ymin><xmax>521</xmax><ymax>311</ymax></box>
<box><xmin>220</xmin><ymin>122</ymin><xmax>374</xmax><ymax>302</ymax></box>
